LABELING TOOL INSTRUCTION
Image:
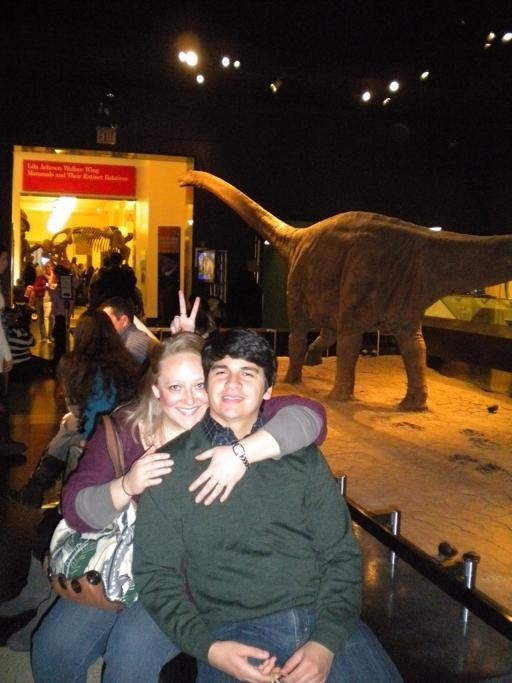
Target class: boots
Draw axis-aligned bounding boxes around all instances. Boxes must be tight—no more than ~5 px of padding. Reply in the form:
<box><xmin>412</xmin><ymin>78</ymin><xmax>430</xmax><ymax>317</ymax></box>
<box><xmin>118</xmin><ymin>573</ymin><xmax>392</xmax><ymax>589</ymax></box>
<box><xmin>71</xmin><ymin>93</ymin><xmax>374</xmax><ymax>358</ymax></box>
<box><xmin>5</xmin><ymin>454</ymin><xmax>68</xmax><ymax>517</ymax></box>
<box><xmin>0</xmin><ymin>550</ymin><xmax>53</xmax><ymax>618</ymax></box>
<box><xmin>5</xmin><ymin>586</ymin><xmax>62</xmax><ymax>652</ymax></box>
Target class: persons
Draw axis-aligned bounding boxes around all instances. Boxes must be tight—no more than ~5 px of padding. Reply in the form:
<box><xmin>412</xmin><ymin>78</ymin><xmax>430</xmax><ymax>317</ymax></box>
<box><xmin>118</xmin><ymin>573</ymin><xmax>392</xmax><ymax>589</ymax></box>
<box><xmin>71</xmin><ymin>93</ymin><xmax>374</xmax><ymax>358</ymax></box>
<box><xmin>30</xmin><ymin>333</ymin><xmax>325</xmax><ymax>683</ymax></box>
<box><xmin>1</xmin><ymin>230</ymin><xmax>202</xmax><ymax>654</ymax></box>
<box><xmin>134</xmin><ymin>330</ymin><xmax>411</xmax><ymax>683</ymax></box>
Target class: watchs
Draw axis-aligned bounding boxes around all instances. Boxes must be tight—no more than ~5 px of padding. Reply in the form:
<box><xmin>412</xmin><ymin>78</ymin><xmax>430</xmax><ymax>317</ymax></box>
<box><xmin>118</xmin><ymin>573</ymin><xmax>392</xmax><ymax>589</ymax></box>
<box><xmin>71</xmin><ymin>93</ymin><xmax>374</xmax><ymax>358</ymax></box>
<box><xmin>231</xmin><ymin>439</ymin><xmax>250</xmax><ymax>469</ymax></box>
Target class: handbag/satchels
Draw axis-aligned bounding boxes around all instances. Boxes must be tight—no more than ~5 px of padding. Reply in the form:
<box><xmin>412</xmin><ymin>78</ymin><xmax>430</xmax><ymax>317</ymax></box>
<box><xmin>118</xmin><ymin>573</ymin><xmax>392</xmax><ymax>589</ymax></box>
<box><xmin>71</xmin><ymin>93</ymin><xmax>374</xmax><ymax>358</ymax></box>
<box><xmin>48</xmin><ymin>493</ymin><xmax>140</xmax><ymax>611</ymax></box>
